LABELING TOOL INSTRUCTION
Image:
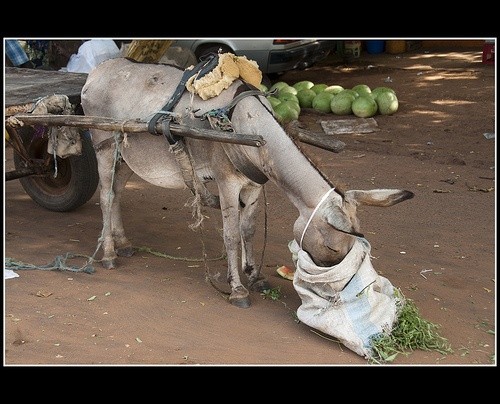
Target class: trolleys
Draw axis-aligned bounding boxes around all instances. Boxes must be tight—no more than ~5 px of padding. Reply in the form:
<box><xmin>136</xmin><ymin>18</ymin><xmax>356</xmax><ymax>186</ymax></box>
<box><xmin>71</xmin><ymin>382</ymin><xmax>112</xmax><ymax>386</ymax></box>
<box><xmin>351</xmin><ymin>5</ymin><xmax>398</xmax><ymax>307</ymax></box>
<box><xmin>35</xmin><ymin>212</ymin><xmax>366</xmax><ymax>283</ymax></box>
<box><xmin>7</xmin><ymin>37</ymin><xmax>348</xmax><ymax>210</ymax></box>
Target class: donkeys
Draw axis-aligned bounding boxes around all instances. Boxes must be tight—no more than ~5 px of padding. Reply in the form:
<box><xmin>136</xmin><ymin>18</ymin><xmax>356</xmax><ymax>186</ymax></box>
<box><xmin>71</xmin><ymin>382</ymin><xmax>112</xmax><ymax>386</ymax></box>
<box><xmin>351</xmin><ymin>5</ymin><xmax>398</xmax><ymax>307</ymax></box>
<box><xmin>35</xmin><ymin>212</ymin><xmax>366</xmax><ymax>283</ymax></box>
<box><xmin>81</xmin><ymin>56</ymin><xmax>415</xmax><ymax>308</ymax></box>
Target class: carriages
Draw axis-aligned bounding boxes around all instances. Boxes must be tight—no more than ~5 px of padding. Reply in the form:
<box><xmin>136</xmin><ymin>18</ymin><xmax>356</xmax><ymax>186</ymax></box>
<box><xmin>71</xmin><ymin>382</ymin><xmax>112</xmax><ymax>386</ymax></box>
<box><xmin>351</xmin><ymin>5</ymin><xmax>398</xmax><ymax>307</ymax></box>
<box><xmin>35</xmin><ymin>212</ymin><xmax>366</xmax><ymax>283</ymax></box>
<box><xmin>5</xmin><ymin>68</ymin><xmax>415</xmax><ymax>310</ymax></box>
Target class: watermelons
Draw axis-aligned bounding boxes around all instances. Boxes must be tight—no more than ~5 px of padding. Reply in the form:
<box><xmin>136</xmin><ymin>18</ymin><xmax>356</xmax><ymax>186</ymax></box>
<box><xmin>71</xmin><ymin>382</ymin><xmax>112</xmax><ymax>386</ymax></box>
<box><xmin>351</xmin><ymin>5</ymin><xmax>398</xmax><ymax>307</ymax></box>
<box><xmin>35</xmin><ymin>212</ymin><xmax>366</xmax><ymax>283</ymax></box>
<box><xmin>260</xmin><ymin>80</ymin><xmax>399</xmax><ymax>122</ymax></box>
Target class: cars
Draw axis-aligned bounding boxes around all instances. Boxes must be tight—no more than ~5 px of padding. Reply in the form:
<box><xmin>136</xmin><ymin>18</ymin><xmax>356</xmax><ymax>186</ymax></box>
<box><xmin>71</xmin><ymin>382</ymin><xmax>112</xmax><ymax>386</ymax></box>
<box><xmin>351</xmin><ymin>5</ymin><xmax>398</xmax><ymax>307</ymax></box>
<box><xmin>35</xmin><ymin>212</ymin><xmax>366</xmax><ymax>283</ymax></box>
<box><xmin>156</xmin><ymin>37</ymin><xmax>336</xmax><ymax>81</ymax></box>
